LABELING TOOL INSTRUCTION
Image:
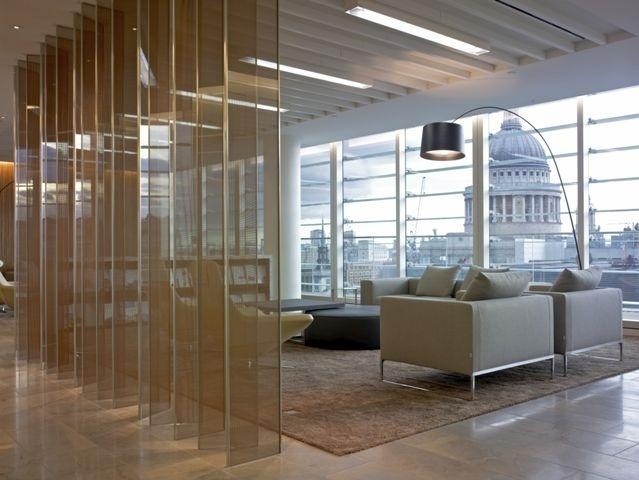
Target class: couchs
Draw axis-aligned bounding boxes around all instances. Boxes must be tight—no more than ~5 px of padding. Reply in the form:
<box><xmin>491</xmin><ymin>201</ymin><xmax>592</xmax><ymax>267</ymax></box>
<box><xmin>361</xmin><ymin>277</ymin><xmax>553</xmax><ymax>306</ymax></box>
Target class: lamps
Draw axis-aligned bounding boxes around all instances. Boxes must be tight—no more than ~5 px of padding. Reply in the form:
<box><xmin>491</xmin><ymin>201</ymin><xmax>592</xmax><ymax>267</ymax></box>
<box><xmin>420</xmin><ymin>106</ymin><xmax>583</xmax><ymax>270</ymax></box>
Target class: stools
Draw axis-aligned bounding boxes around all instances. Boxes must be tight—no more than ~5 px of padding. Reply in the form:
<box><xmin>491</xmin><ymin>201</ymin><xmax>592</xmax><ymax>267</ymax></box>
<box><xmin>303</xmin><ymin>303</ymin><xmax>381</xmax><ymax>351</ymax></box>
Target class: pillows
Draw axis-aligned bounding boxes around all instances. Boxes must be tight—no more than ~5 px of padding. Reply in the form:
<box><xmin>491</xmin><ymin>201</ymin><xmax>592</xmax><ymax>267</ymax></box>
<box><xmin>549</xmin><ymin>266</ymin><xmax>602</xmax><ymax>292</ymax></box>
<box><xmin>416</xmin><ymin>264</ymin><xmax>533</xmax><ymax>302</ymax></box>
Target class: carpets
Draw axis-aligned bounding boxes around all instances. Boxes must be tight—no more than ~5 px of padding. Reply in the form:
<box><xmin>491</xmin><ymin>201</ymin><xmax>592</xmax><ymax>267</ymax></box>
<box><xmin>67</xmin><ymin>321</ymin><xmax>638</xmax><ymax>457</ymax></box>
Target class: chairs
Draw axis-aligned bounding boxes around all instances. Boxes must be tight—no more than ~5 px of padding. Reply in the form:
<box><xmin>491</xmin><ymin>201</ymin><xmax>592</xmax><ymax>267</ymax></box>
<box><xmin>158</xmin><ymin>264</ymin><xmax>314</xmax><ymax>380</ymax></box>
<box><xmin>0</xmin><ymin>272</ymin><xmax>24</xmax><ymax>317</ymax></box>
<box><xmin>379</xmin><ymin>287</ymin><xmax>625</xmax><ymax>400</ymax></box>
